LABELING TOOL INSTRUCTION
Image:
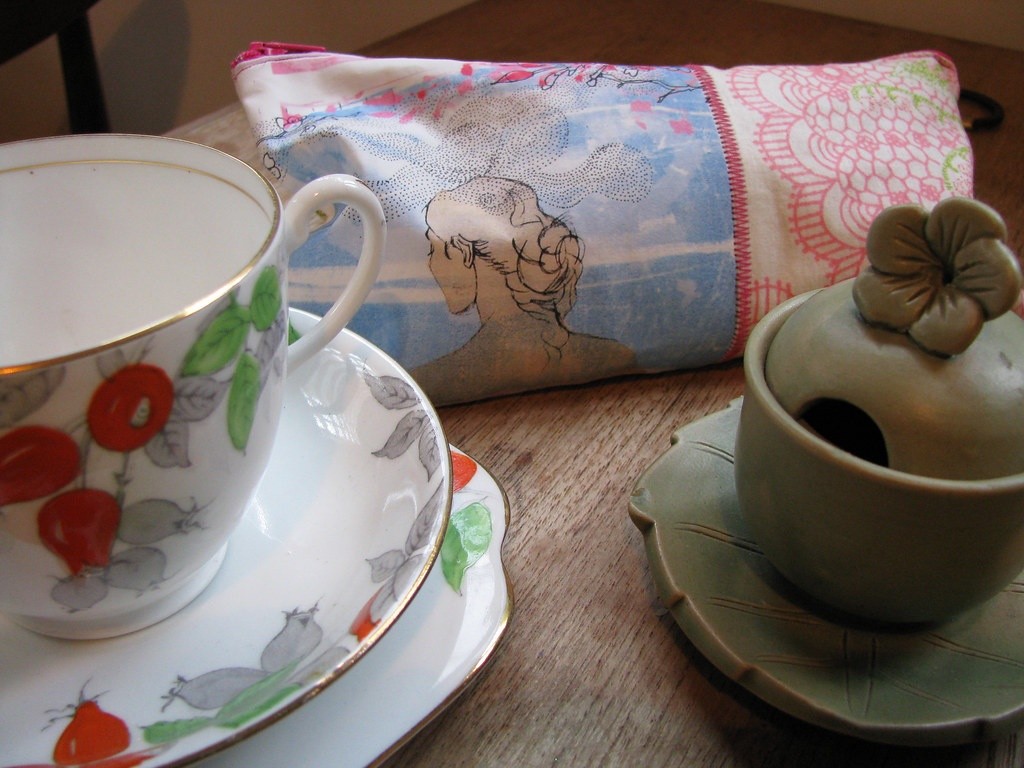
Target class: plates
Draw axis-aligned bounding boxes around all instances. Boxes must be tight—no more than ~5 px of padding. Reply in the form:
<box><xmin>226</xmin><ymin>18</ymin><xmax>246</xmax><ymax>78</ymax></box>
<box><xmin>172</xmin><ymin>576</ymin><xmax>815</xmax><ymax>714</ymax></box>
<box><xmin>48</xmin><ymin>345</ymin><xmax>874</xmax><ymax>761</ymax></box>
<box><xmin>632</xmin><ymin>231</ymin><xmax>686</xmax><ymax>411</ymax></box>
<box><xmin>186</xmin><ymin>443</ymin><xmax>517</xmax><ymax>768</ymax></box>
<box><xmin>626</xmin><ymin>394</ymin><xmax>1023</xmax><ymax>745</ymax></box>
<box><xmin>0</xmin><ymin>305</ymin><xmax>452</xmax><ymax>768</ymax></box>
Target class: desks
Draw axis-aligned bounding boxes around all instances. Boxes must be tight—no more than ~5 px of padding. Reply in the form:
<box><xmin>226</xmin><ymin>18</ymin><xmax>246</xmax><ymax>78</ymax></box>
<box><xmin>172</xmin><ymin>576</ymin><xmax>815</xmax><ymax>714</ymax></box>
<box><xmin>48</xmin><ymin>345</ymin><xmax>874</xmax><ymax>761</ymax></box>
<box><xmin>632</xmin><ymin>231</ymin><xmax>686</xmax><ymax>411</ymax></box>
<box><xmin>152</xmin><ymin>0</ymin><xmax>1024</xmax><ymax>768</ymax></box>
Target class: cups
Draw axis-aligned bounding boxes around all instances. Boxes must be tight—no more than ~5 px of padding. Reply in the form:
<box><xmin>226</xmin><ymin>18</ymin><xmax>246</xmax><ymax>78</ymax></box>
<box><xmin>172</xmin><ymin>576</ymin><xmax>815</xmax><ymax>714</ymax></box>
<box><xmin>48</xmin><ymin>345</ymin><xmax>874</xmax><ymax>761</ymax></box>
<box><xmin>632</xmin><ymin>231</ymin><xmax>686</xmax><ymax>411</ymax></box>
<box><xmin>1</xmin><ymin>133</ymin><xmax>386</xmax><ymax>641</ymax></box>
<box><xmin>733</xmin><ymin>198</ymin><xmax>1024</xmax><ymax>630</ymax></box>
